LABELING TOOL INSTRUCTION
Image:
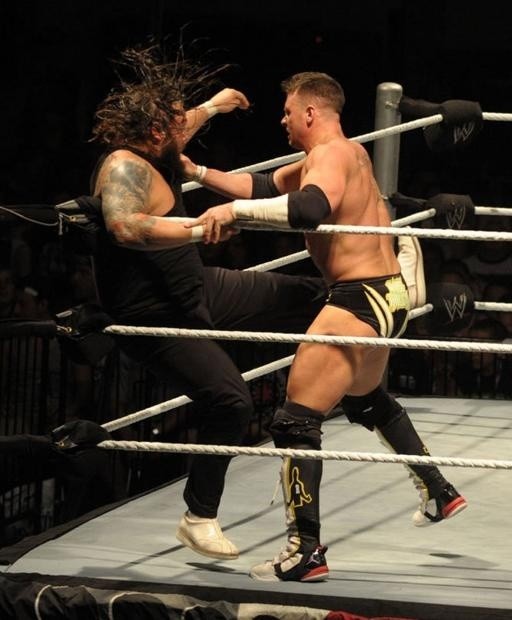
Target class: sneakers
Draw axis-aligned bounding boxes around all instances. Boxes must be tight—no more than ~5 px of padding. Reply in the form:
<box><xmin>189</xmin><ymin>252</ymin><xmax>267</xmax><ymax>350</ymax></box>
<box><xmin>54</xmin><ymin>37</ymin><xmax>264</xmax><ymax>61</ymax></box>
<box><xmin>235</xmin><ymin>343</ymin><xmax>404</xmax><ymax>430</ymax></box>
<box><xmin>396</xmin><ymin>227</ymin><xmax>427</xmax><ymax>308</ymax></box>
<box><xmin>249</xmin><ymin>545</ymin><xmax>329</xmax><ymax>582</ymax></box>
<box><xmin>412</xmin><ymin>486</ymin><xmax>467</xmax><ymax>527</ymax></box>
<box><xmin>176</xmin><ymin>513</ymin><xmax>239</xmax><ymax>561</ymax></box>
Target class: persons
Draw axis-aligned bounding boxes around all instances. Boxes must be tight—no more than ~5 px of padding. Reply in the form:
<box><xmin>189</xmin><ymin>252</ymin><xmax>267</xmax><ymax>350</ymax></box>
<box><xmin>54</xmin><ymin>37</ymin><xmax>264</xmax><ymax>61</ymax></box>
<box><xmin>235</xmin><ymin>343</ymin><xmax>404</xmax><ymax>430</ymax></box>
<box><xmin>179</xmin><ymin>72</ymin><xmax>468</xmax><ymax>582</ymax></box>
<box><xmin>92</xmin><ymin>49</ymin><xmax>427</xmax><ymax>558</ymax></box>
<box><xmin>2</xmin><ymin>209</ymin><xmax>512</xmax><ymax>492</ymax></box>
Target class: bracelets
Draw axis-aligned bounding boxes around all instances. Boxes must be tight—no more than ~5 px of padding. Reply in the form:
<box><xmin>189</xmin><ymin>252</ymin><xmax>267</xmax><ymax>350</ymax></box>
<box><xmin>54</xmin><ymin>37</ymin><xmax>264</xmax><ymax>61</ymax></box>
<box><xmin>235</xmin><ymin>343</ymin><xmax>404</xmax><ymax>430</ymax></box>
<box><xmin>200</xmin><ymin>101</ymin><xmax>218</xmax><ymax>117</ymax></box>
<box><xmin>193</xmin><ymin>165</ymin><xmax>208</xmax><ymax>183</ymax></box>
